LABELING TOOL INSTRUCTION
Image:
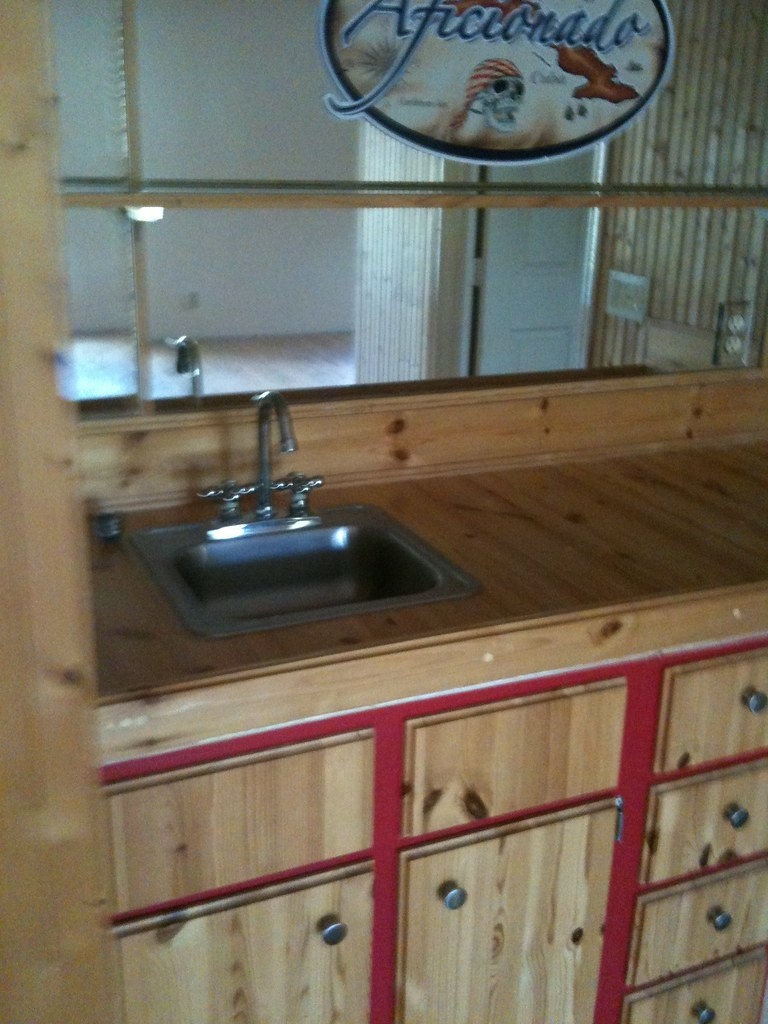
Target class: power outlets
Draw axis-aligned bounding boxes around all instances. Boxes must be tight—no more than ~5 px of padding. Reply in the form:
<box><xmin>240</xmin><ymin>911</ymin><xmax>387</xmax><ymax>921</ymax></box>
<box><xmin>713</xmin><ymin>301</ymin><xmax>749</xmax><ymax>365</ymax></box>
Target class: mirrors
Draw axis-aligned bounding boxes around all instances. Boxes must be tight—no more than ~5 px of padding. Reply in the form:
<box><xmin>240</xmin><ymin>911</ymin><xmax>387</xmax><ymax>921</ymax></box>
<box><xmin>45</xmin><ymin>0</ymin><xmax>768</xmax><ymax>424</ymax></box>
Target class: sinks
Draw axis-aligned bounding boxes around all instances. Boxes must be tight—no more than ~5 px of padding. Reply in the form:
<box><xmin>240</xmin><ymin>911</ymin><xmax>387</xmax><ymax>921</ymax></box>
<box><xmin>128</xmin><ymin>502</ymin><xmax>482</xmax><ymax>639</ymax></box>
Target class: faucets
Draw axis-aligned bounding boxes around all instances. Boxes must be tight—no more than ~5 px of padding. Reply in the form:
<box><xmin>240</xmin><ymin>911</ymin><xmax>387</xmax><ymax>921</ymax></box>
<box><xmin>175</xmin><ymin>335</ymin><xmax>205</xmax><ymax>397</ymax></box>
<box><xmin>195</xmin><ymin>389</ymin><xmax>327</xmax><ymax>541</ymax></box>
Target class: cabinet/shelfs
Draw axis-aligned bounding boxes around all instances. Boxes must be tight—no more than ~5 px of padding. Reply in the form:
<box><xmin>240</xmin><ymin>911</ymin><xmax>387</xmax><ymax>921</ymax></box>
<box><xmin>89</xmin><ymin>627</ymin><xmax>768</xmax><ymax>1024</ymax></box>
